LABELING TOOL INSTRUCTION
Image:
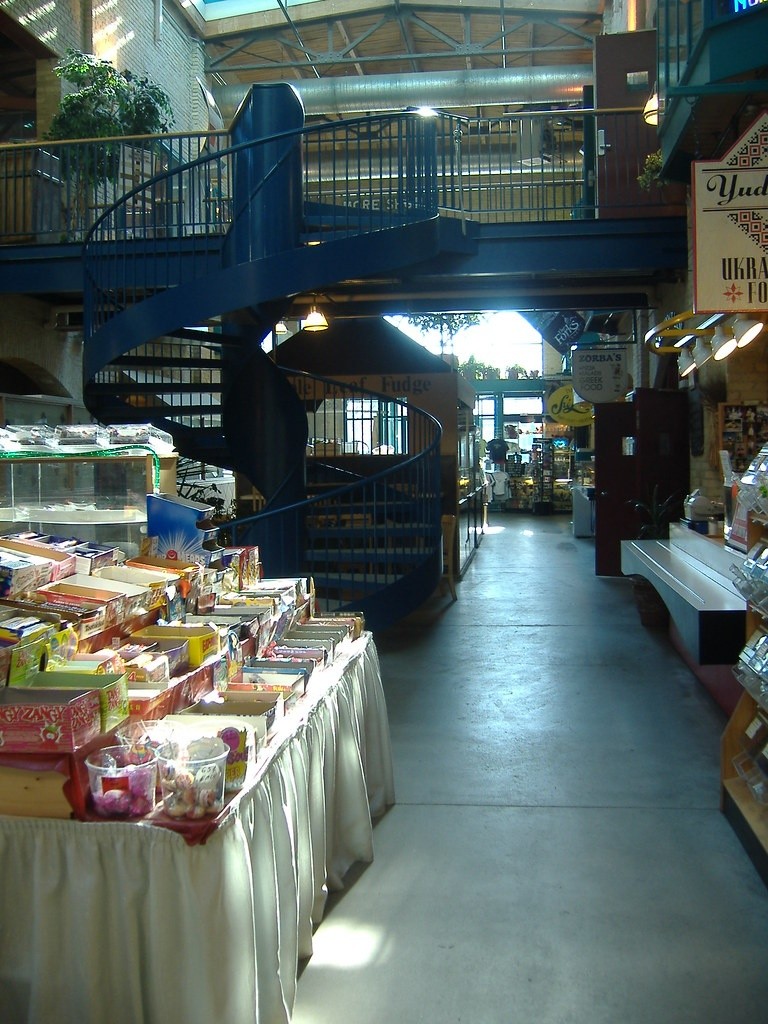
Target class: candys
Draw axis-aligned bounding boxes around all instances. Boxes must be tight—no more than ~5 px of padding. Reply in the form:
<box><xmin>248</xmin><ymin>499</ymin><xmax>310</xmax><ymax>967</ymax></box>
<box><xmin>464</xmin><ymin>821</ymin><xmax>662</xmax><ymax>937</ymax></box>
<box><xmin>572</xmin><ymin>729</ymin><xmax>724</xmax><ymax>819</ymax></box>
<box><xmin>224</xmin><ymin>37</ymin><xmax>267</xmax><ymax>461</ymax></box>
<box><xmin>90</xmin><ymin>719</ymin><xmax>223</xmax><ymax>819</ymax></box>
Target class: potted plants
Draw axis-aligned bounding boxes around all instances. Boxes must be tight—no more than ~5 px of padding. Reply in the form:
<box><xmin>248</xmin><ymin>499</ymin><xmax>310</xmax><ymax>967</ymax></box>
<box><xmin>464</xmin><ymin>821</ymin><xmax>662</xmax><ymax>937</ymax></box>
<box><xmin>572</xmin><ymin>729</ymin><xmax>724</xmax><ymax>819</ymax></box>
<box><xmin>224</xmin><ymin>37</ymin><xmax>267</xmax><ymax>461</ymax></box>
<box><xmin>626</xmin><ymin>484</ymin><xmax>677</xmax><ymax>627</ymax></box>
<box><xmin>457</xmin><ymin>354</ymin><xmax>528</xmax><ymax>379</ymax></box>
<box><xmin>24</xmin><ymin>47</ymin><xmax>177</xmax><ymax>242</ymax></box>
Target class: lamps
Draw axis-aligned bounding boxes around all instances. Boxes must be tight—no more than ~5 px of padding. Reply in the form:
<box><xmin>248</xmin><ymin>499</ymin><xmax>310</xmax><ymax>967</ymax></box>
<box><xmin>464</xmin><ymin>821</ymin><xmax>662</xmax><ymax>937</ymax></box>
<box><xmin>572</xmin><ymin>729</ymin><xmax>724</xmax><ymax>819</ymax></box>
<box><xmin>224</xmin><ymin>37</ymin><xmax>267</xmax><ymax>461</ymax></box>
<box><xmin>641</xmin><ymin>80</ymin><xmax>658</xmax><ymax>126</ymax></box>
<box><xmin>304</xmin><ymin>295</ymin><xmax>329</xmax><ymax>332</ymax></box>
<box><xmin>276</xmin><ymin>321</ymin><xmax>288</xmax><ymax>334</ymax></box>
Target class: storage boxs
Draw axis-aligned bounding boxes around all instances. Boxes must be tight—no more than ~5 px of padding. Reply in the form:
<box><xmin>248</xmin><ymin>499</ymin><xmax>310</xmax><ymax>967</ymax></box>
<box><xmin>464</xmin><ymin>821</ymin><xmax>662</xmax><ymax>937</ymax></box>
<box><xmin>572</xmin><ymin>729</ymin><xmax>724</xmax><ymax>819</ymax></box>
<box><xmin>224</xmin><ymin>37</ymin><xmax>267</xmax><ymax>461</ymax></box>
<box><xmin>0</xmin><ymin>491</ymin><xmax>366</xmax><ymax>764</ymax></box>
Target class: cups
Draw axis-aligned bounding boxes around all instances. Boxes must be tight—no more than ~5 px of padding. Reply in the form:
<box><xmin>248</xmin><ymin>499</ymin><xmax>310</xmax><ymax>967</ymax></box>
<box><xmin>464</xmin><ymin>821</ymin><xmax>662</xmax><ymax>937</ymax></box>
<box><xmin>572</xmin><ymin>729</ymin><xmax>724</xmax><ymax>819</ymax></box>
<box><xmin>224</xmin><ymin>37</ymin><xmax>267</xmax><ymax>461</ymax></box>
<box><xmin>157</xmin><ymin>738</ymin><xmax>229</xmax><ymax>816</ymax></box>
<box><xmin>117</xmin><ymin>721</ymin><xmax>187</xmax><ymax>799</ymax></box>
<box><xmin>85</xmin><ymin>747</ymin><xmax>159</xmax><ymax>818</ymax></box>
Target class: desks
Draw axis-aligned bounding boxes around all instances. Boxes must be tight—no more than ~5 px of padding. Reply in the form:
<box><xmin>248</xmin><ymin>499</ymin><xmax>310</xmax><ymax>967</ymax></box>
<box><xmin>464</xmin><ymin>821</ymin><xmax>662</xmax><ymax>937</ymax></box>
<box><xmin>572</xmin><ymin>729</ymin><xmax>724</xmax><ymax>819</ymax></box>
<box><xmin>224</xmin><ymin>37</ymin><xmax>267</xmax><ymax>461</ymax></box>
<box><xmin>0</xmin><ymin>630</ymin><xmax>397</xmax><ymax>1024</ymax></box>
